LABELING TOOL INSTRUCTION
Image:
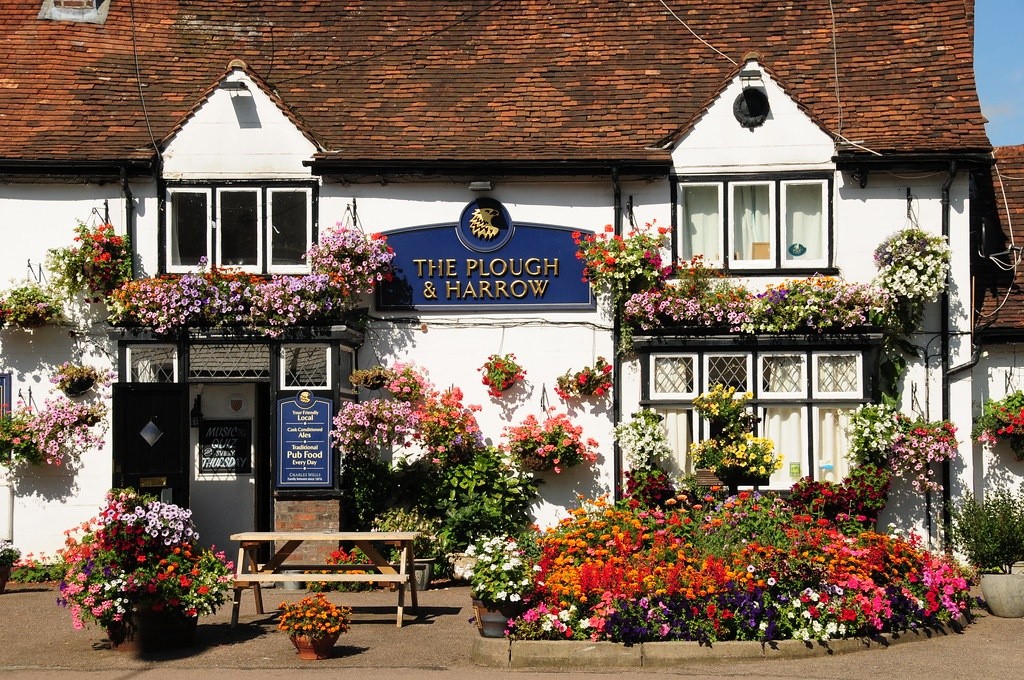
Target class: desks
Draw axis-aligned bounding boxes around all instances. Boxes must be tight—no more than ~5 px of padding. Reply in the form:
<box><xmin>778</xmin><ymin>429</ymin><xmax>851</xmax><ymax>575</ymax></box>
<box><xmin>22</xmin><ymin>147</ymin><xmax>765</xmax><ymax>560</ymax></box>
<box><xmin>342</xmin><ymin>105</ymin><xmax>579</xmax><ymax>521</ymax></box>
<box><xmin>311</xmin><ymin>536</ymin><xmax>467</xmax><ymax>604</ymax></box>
<box><xmin>230</xmin><ymin>531</ymin><xmax>423</xmax><ymax>628</ymax></box>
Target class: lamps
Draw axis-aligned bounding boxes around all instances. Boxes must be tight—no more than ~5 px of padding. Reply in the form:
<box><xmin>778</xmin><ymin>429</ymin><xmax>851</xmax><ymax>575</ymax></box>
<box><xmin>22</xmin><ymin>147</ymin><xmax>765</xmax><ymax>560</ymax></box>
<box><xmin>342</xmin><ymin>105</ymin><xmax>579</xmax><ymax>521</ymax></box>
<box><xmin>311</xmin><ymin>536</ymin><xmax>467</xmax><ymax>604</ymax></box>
<box><xmin>380</xmin><ymin>178</ymin><xmax>388</xmax><ymax>186</ymax></box>
<box><xmin>341</xmin><ymin>178</ymin><xmax>348</xmax><ymax>186</ymax></box>
<box><xmin>851</xmin><ymin>169</ymin><xmax>870</xmax><ymax>189</ymax></box>
<box><xmin>218</xmin><ymin>81</ymin><xmax>253</xmax><ymax>97</ymax></box>
<box><xmin>739</xmin><ymin>69</ymin><xmax>762</xmax><ymax>80</ymax></box>
<box><xmin>469</xmin><ymin>178</ymin><xmax>492</xmax><ymax>192</ymax></box>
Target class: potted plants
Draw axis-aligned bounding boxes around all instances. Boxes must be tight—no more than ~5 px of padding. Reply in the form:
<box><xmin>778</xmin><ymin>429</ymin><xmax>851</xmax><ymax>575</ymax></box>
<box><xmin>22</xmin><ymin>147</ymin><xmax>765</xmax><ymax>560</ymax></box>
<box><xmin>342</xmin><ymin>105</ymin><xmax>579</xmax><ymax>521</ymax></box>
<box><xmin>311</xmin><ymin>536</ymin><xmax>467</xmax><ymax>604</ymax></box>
<box><xmin>936</xmin><ymin>484</ymin><xmax>1024</xmax><ymax>617</ymax></box>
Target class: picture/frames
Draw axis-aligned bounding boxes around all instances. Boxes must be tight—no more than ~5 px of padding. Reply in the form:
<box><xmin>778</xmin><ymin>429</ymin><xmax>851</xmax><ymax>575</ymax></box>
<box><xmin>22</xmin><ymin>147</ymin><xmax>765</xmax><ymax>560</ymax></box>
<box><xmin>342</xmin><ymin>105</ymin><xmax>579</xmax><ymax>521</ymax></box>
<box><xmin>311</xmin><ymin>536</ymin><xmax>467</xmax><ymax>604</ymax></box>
<box><xmin>752</xmin><ymin>242</ymin><xmax>770</xmax><ymax>260</ymax></box>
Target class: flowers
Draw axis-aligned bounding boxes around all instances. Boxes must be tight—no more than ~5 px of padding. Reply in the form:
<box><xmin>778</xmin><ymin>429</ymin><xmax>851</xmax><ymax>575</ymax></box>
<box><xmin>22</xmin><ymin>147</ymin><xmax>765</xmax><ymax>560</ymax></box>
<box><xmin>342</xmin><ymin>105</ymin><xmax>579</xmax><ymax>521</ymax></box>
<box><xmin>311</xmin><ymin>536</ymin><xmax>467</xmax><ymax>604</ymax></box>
<box><xmin>0</xmin><ymin>218</ymin><xmax>1024</xmax><ymax>646</ymax></box>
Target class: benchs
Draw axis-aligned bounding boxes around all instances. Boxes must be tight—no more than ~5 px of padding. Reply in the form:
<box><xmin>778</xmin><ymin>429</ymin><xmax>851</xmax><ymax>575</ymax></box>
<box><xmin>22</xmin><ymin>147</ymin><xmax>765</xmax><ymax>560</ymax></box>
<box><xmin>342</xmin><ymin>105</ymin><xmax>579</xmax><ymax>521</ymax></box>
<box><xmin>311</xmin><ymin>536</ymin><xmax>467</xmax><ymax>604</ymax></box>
<box><xmin>223</xmin><ymin>556</ymin><xmax>427</xmax><ymax>585</ymax></box>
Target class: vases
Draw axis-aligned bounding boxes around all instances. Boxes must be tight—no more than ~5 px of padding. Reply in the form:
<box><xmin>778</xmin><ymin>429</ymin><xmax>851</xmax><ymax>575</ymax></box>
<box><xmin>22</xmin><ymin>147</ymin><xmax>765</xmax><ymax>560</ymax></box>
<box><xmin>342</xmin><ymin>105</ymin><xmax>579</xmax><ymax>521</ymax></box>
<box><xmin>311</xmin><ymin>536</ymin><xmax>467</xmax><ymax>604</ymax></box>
<box><xmin>470</xmin><ymin>592</ymin><xmax>522</xmax><ymax>639</ymax></box>
<box><xmin>286</xmin><ymin>628</ymin><xmax>341</xmax><ymax>660</ymax></box>
<box><xmin>0</xmin><ymin>563</ymin><xmax>13</xmax><ymax>595</ymax></box>
<box><xmin>579</xmin><ymin>374</ymin><xmax>610</xmax><ymax>396</ymax></box>
<box><xmin>520</xmin><ymin>441</ymin><xmax>559</xmax><ymax>473</ymax></box>
<box><xmin>616</xmin><ymin>261</ymin><xmax>651</xmax><ymax>295</ymax></box>
<box><xmin>105</xmin><ymin>602</ymin><xmax>139</xmax><ymax>650</ymax></box>
<box><xmin>710</xmin><ymin>415</ymin><xmax>738</xmax><ymax>439</ymax></box>
<box><xmin>60</xmin><ymin>373</ymin><xmax>95</xmax><ymax>395</ymax></box>
